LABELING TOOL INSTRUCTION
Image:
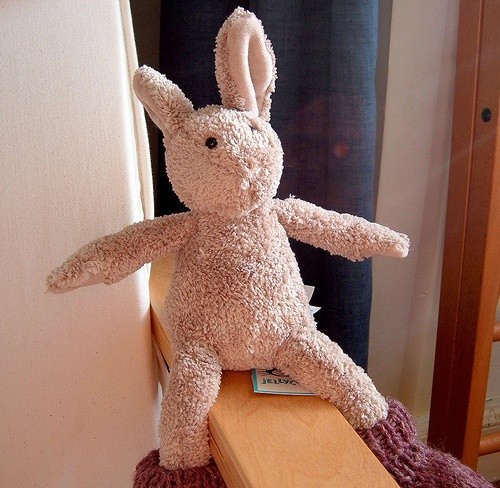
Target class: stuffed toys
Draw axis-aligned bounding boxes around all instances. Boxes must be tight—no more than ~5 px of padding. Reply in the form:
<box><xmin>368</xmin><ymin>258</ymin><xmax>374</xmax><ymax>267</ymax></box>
<box><xmin>47</xmin><ymin>5</ymin><xmax>495</xmax><ymax>488</ymax></box>
